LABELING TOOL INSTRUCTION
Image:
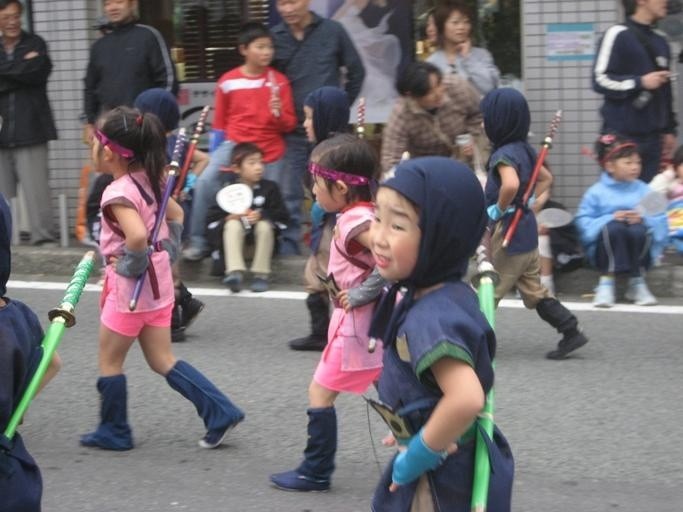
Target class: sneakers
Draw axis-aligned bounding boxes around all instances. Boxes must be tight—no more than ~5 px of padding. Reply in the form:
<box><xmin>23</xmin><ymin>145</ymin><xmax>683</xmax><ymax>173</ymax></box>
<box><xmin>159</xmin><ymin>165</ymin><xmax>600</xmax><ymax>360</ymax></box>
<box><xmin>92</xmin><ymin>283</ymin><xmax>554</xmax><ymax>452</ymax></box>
<box><xmin>623</xmin><ymin>277</ymin><xmax>656</xmax><ymax>307</ymax></box>
<box><xmin>268</xmin><ymin>470</ymin><xmax>330</xmax><ymax>492</ymax></box>
<box><xmin>172</xmin><ymin>296</ymin><xmax>202</xmax><ymax>342</ymax></box>
<box><xmin>547</xmin><ymin>338</ymin><xmax>589</xmax><ymax>360</ymax></box>
<box><xmin>198</xmin><ymin>415</ymin><xmax>241</xmax><ymax>449</ymax></box>
<box><xmin>593</xmin><ymin>275</ymin><xmax>619</xmax><ymax>311</ymax></box>
<box><xmin>78</xmin><ymin>433</ymin><xmax>96</xmax><ymax>446</ymax></box>
<box><xmin>287</xmin><ymin>333</ymin><xmax>326</xmax><ymax>352</ymax></box>
<box><xmin>250</xmin><ymin>276</ymin><xmax>270</xmax><ymax>293</ymax></box>
<box><xmin>232</xmin><ymin>274</ymin><xmax>245</xmax><ymax>294</ymax></box>
<box><xmin>180</xmin><ymin>243</ymin><xmax>208</xmax><ymax>262</ymax></box>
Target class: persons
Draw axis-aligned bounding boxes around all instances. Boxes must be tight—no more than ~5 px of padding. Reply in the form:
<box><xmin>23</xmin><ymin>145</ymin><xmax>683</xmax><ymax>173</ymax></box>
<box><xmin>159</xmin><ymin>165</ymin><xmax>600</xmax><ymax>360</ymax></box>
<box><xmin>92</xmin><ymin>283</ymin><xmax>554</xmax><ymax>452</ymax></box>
<box><xmin>288</xmin><ymin>87</ymin><xmax>350</xmax><ymax>352</ymax></box>
<box><xmin>1</xmin><ymin>195</ymin><xmax>60</xmax><ymax>512</ymax></box>
<box><xmin>0</xmin><ymin>1</ymin><xmax>57</xmax><ymax>248</ymax></box>
<box><xmin>80</xmin><ymin>106</ymin><xmax>242</xmax><ymax>450</ymax></box>
<box><xmin>464</xmin><ymin>87</ymin><xmax>590</xmax><ymax>358</ymax></box>
<box><xmin>84</xmin><ymin>0</ymin><xmax>550</xmax><ymax>345</ymax></box>
<box><xmin>366</xmin><ymin>156</ymin><xmax>517</xmax><ymax>512</ymax></box>
<box><xmin>575</xmin><ymin>0</ymin><xmax>682</xmax><ymax>308</ymax></box>
<box><xmin>266</xmin><ymin>133</ymin><xmax>405</xmax><ymax>491</ymax></box>
